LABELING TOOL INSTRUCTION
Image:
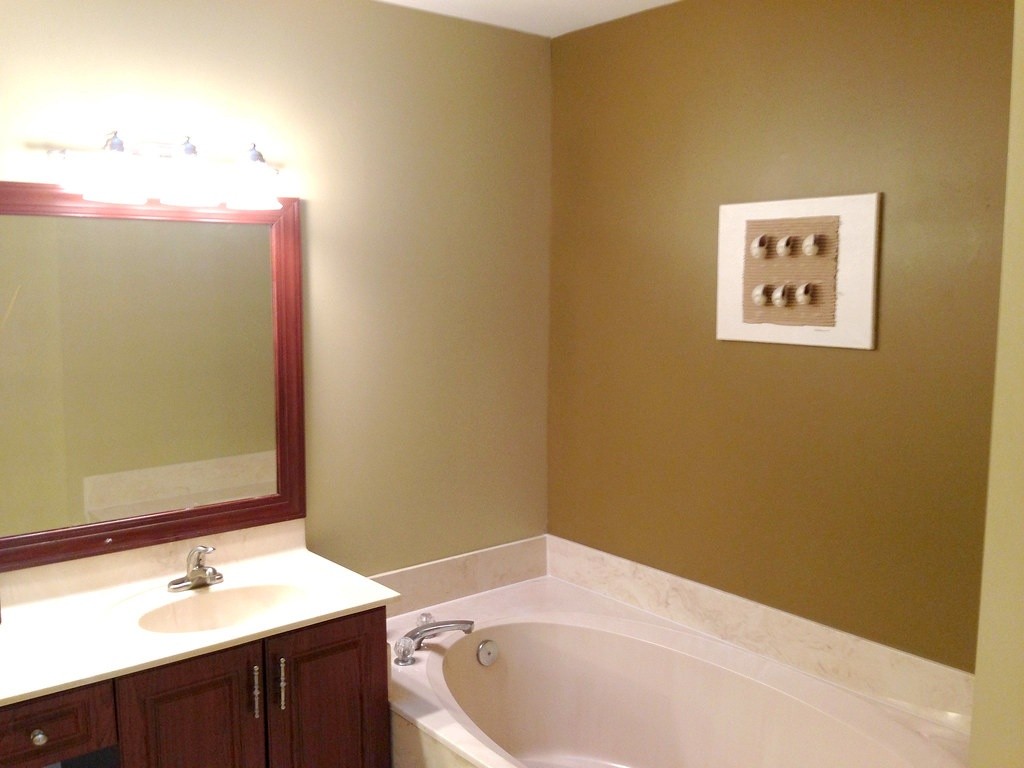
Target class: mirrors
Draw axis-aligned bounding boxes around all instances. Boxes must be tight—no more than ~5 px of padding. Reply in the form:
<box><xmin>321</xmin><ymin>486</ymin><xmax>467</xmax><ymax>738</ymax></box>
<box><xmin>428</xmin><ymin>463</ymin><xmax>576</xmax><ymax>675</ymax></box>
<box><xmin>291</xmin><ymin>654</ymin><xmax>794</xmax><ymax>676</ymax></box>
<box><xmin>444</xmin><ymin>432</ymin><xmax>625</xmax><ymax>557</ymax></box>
<box><xmin>1</xmin><ymin>181</ymin><xmax>308</xmax><ymax>577</ymax></box>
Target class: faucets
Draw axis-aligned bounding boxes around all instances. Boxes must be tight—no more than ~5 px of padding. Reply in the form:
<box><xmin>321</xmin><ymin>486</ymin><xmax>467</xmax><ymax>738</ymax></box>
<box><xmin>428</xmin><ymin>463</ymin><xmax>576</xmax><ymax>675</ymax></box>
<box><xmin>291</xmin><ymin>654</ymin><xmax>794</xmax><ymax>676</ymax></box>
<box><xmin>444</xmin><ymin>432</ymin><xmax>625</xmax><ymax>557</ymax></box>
<box><xmin>403</xmin><ymin>619</ymin><xmax>475</xmax><ymax>651</ymax></box>
<box><xmin>167</xmin><ymin>545</ymin><xmax>224</xmax><ymax>593</ymax></box>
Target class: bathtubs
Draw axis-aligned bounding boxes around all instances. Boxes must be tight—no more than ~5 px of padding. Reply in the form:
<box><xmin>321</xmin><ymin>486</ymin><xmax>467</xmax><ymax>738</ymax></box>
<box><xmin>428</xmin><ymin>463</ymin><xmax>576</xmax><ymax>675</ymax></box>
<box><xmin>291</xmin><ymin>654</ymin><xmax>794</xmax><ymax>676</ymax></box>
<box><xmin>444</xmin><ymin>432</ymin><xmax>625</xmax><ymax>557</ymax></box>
<box><xmin>382</xmin><ymin>576</ymin><xmax>970</xmax><ymax>768</ymax></box>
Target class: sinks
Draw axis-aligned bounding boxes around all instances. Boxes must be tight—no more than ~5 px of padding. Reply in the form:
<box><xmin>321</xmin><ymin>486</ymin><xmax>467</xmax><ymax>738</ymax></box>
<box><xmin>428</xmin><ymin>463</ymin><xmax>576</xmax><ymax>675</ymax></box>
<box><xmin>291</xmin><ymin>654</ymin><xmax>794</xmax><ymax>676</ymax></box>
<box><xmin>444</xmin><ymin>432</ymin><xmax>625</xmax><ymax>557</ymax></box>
<box><xmin>137</xmin><ymin>581</ymin><xmax>299</xmax><ymax>636</ymax></box>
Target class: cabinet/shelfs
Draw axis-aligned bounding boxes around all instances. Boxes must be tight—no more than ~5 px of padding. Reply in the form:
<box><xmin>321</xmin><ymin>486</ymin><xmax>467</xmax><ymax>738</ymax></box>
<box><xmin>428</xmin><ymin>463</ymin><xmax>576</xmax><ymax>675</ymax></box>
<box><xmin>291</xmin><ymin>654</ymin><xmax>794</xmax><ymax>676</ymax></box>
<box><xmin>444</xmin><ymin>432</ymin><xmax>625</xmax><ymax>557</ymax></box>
<box><xmin>0</xmin><ymin>606</ymin><xmax>394</xmax><ymax>768</ymax></box>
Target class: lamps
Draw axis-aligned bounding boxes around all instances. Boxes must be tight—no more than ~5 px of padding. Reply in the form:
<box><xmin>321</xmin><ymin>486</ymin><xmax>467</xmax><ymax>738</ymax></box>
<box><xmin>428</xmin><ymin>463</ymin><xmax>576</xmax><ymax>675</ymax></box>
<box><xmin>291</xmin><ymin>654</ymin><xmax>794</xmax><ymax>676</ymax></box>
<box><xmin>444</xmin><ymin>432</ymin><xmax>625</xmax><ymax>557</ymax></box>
<box><xmin>22</xmin><ymin>129</ymin><xmax>287</xmax><ymax>212</ymax></box>
